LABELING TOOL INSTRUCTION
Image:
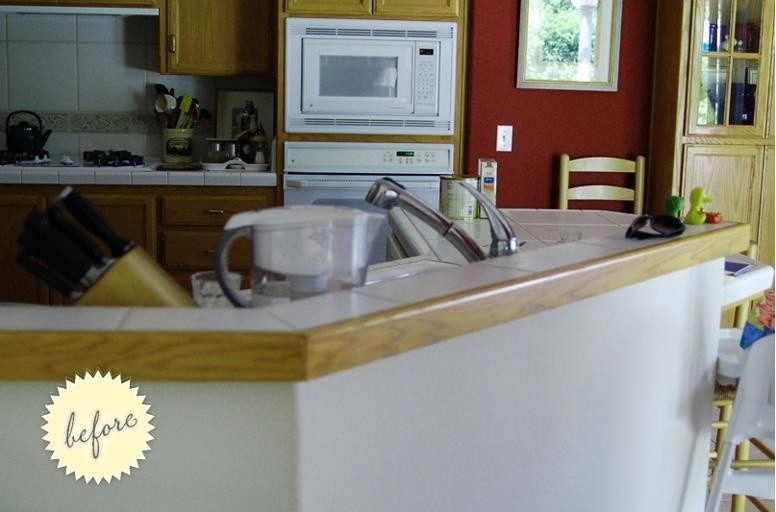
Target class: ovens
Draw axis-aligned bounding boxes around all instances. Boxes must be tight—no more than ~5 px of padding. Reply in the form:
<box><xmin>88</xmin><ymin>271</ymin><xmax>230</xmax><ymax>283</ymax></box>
<box><xmin>282</xmin><ymin>143</ymin><xmax>453</xmax><ymax>295</ymax></box>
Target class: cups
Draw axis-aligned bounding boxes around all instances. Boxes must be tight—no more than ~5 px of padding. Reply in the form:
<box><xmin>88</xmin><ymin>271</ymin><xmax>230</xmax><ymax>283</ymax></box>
<box><xmin>192</xmin><ymin>272</ymin><xmax>242</xmax><ymax>311</ymax></box>
<box><xmin>162</xmin><ymin>127</ymin><xmax>195</xmax><ymax>166</ymax></box>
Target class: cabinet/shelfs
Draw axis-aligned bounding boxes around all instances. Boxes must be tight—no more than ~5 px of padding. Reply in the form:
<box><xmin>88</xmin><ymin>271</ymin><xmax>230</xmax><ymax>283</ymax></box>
<box><xmin>647</xmin><ymin>0</ymin><xmax>775</xmax><ymax>264</ymax></box>
<box><xmin>158</xmin><ymin>0</ymin><xmax>278</xmax><ymax>79</ymax></box>
<box><xmin>283</xmin><ymin>0</ymin><xmax>461</xmax><ymax>18</ymax></box>
<box><xmin>0</xmin><ymin>185</ymin><xmax>274</xmax><ymax>302</ymax></box>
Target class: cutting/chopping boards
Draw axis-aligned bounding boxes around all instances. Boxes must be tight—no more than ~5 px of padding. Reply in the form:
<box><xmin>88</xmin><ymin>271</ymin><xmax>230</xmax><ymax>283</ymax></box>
<box><xmin>216</xmin><ymin>91</ymin><xmax>274</xmax><ymax>144</ymax></box>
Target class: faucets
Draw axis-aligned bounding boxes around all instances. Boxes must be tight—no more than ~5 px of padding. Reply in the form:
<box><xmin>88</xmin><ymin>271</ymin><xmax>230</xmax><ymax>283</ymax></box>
<box><xmin>366</xmin><ymin>177</ymin><xmax>517</xmax><ymax>259</ymax></box>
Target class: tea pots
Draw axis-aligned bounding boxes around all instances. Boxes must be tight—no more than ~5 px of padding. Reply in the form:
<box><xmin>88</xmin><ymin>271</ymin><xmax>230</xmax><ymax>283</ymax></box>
<box><xmin>4</xmin><ymin>110</ymin><xmax>53</xmax><ymax>153</ymax></box>
<box><xmin>216</xmin><ymin>204</ymin><xmax>385</xmax><ymax>311</ymax></box>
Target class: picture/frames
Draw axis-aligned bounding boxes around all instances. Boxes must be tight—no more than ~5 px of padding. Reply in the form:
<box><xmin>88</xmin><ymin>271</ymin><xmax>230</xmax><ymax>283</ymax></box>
<box><xmin>516</xmin><ymin>0</ymin><xmax>622</xmax><ymax>92</ymax></box>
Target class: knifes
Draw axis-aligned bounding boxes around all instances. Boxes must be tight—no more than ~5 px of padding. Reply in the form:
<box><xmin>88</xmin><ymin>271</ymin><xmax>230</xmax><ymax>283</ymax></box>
<box><xmin>14</xmin><ymin>186</ymin><xmax>134</xmax><ymax>302</ymax></box>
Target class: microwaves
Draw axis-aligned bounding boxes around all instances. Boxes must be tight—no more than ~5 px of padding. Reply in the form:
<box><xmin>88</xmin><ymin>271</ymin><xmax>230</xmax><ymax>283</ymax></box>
<box><xmin>285</xmin><ymin>17</ymin><xmax>457</xmax><ymax>138</ymax></box>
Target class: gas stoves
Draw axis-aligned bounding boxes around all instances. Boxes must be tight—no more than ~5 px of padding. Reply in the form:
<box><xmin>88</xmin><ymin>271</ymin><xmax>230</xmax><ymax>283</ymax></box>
<box><xmin>0</xmin><ymin>149</ymin><xmax>161</xmax><ymax>172</ymax></box>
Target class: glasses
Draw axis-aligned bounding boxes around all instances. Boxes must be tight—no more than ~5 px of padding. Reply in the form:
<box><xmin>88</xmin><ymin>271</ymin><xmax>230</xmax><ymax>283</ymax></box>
<box><xmin>625</xmin><ymin>214</ymin><xmax>686</xmax><ymax>239</ymax></box>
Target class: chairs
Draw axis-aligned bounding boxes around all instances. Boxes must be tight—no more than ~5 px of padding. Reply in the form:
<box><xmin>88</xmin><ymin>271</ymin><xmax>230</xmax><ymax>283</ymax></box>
<box><xmin>708</xmin><ymin>335</ymin><xmax>775</xmax><ymax>510</ymax></box>
<box><xmin>709</xmin><ymin>246</ymin><xmax>755</xmax><ymax>511</ymax></box>
<box><xmin>560</xmin><ymin>154</ymin><xmax>646</xmax><ymax>215</ymax></box>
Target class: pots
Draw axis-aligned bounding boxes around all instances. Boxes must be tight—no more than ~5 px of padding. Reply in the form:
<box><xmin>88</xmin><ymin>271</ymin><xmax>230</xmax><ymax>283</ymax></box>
<box><xmin>199</xmin><ymin>130</ymin><xmax>269</xmax><ymax>171</ymax></box>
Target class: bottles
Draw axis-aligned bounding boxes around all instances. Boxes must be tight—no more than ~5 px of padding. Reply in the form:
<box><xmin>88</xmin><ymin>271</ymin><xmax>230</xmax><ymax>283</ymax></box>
<box><xmin>240</xmin><ymin>101</ymin><xmax>266</xmax><ymax>166</ymax></box>
